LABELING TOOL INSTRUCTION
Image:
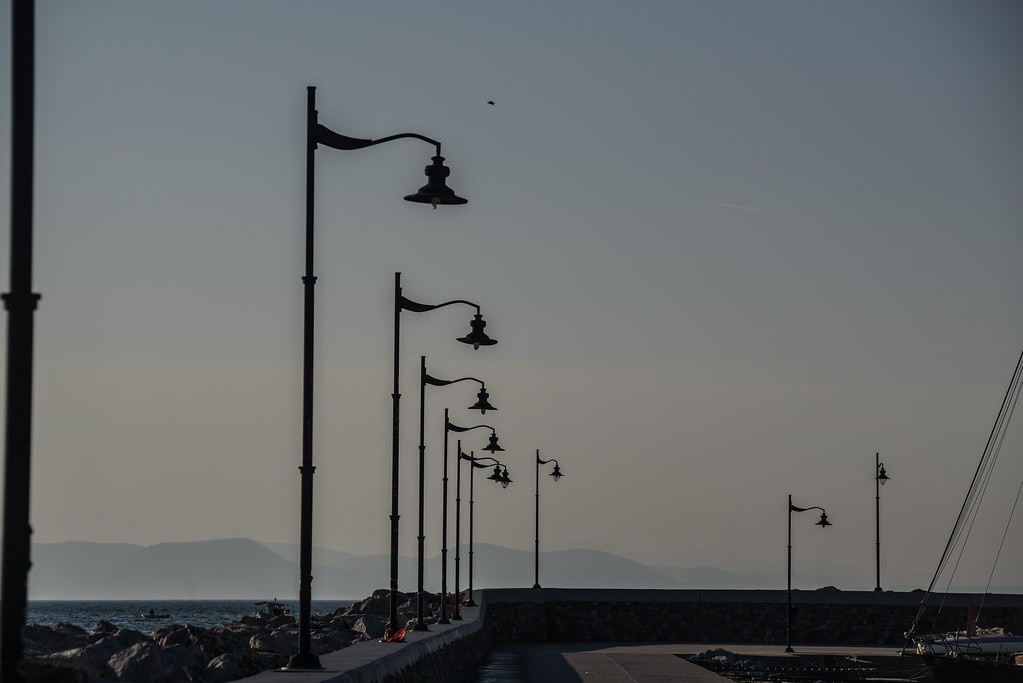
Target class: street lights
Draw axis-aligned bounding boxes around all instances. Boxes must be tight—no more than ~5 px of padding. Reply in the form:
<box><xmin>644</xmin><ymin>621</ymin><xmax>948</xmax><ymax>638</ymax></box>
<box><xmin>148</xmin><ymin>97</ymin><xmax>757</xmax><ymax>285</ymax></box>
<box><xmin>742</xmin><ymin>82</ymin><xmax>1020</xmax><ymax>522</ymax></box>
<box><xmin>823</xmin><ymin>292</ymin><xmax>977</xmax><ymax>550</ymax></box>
<box><xmin>414</xmin><ymin>354</ymin><xmax>499</xmax><ymax>632</ymax></box>
<box><xmin>534</xmin><ymin>449</ymin><xmax>564</xmax><ymax>587</ymax></box>
<box><xmin>465</xmin><ymin>451</ymin><xmax>514</xmax><ymax>608</ymax></box>
<box><xmin>784</xmin><ymin>494</ymin><xmax>833</xmax><ymax>654</ymax></box>
<box><xmin>875</xmin><ymin>452</ymin><xmax>891</xmax><ymax>591</ymax></box>
<box><xmin>274</xmin><ymin>76</ymin><xmax>472</xmax><ymax>673</ymax></box>
<box><xmin>437</xmin><ymin>407</ymin><xmax>504</xmax><ymax>624</ymax></box>
<box><xmin>384</xmin><ymin>271</ymin><xmax>498</xmax><ymax>641</ymax></box>
<box><xmin>453</xmin><ymin>439</ymin><xmax>504</xmax><ymax>620</ymax></box>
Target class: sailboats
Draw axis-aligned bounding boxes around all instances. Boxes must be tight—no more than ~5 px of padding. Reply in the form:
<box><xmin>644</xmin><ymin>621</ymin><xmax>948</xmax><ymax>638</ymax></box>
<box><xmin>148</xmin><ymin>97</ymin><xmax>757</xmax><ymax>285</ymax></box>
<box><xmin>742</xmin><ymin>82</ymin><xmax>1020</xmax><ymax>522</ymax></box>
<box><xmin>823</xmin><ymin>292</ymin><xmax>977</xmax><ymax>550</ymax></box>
<box><xmin>896</xmin><ymin>349</ymin><xmax>1022</xmax><ymax>683</ymax></box>
